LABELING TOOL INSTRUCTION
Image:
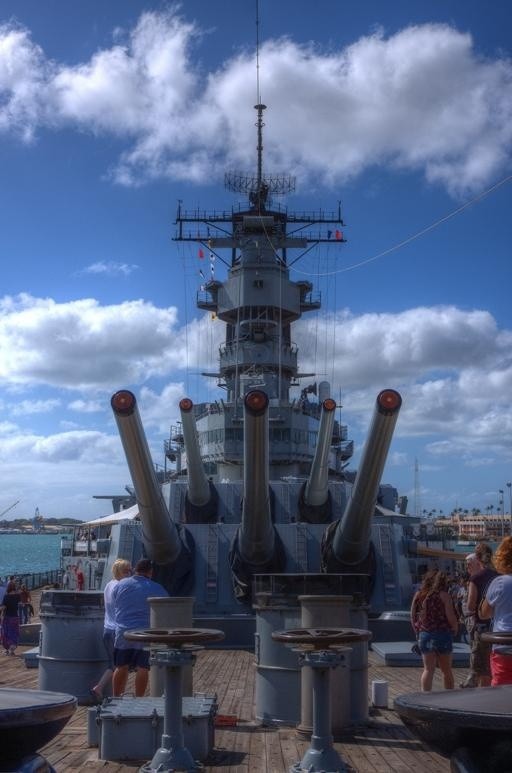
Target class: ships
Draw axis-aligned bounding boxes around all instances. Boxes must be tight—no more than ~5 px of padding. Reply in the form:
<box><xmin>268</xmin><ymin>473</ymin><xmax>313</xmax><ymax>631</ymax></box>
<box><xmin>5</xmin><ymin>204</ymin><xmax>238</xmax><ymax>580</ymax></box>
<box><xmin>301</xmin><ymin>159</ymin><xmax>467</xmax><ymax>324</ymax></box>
<box><xmin>2</xmin><ymin>1</ymin><xmax>510</xmax><ymax>773</ymax></box>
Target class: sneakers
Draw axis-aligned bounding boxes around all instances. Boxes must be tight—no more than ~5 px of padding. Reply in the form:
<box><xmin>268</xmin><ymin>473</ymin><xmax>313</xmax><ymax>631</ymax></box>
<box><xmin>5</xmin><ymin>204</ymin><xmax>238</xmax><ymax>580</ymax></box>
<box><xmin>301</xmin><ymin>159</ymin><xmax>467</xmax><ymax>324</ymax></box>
<box><xmin>3</xmin><ymin>651</ymin><xmax>15</xmax><ymax>656</ymax></box>
<box><xmin>90</xmin><ymin>686</ymin><xmax>104</xmax><ymax>702</ymax></box>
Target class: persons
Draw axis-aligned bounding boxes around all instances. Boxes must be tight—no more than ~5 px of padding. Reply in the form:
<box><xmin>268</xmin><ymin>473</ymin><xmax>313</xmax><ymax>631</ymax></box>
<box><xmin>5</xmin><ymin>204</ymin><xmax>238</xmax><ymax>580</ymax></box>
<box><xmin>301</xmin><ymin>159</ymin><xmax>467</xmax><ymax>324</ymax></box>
<box><xmin>0</xmin><ymin>576</ymin><xmax>33</xmax><ymax>657</ymax></box>
<box><xmin>110</xmin><ymin>559</ymin><xmax>172</xmax><ymax>697</ymax></box>
<box><xmin>88</xmin><ymin>557</ymin><xmax>132</xmax><ymax>702</ymax></box>
<box><xmin>73</xmin><ymin>531</ymin><xmax>95</xmax><ymax>542</ymax></box>
<box><xmin>62</xmin><ymin>563</ymin><xmax>84</xmax><ymax>589</ymax></box>
<box><xmin>409</xmin><ymin>535</ymin><xmax>512</xmax><ymax>692</ymax></box>
